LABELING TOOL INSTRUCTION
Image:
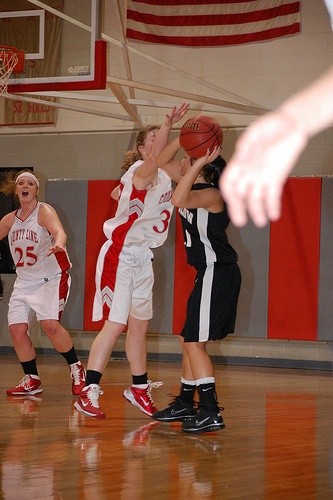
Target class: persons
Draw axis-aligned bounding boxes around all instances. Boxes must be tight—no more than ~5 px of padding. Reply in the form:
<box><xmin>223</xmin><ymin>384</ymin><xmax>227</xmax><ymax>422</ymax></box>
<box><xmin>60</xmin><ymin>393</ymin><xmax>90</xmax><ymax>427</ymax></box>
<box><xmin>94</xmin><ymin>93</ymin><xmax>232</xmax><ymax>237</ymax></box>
<box><xmin>151</xmin><ymin>135</ymin><xmax>240</xmax><ymax>433</ymax></box>
<box><xmin>0</xmin><ymin>168</ymin><xmax>86</xmax><ymax>397</ymax></box>
<box><xmin>220</xmin><ymin>1</ymin><xmax>333</xmax><ymax>228</ymax></box>
<box><xmin>73</xmin><ymin>103</ymin><xmax>191</xmax><ymax>418</ymax></box>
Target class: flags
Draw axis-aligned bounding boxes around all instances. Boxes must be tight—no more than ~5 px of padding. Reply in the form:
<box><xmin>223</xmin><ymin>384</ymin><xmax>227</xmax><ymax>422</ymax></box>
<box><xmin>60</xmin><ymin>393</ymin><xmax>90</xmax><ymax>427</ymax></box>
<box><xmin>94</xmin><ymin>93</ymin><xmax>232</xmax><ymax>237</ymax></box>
<box><xmin>127</xmin><ymin>0</ymin><xmax>303</xmax><ymax>46</ymax></box>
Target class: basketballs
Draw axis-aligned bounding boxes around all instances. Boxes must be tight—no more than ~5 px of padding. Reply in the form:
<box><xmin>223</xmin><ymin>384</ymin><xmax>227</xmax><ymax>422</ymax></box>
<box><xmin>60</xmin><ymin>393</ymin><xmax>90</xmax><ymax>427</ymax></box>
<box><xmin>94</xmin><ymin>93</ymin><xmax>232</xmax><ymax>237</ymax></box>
<box><xmin>179</xmin><ymin>115</ymin><xmax>224</xmax><ymax>159</ymax></box>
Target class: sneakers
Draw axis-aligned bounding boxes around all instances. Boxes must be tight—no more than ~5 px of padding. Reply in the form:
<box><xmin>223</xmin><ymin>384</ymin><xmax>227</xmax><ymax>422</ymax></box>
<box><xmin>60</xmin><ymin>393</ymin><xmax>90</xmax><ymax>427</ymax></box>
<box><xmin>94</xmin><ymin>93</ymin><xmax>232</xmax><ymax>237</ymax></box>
<box><xmin>70</xmin><ymin>361</ymin><xmax>87</xmax><ymax>396</ymax></box>
<box><xmin>73</xmin><ymin>382</ymin><xmax>106</xmax><ymax>420</ymax></box>
<box><xmin>154</xmin><ymin>393</ymin><xmax>197</xmax><ymax>422</ymax></box>
<box><xmin>181</xmin><ymin>403</ymin><xmax>224</xmax><ymax>434</ymax></box>
<box><xmin>7</xmin><ymin>374</ymin><xmax>43</xmax><ymax>395</ymax></box>
<box><xmin>123</xmin><ymin>380</ymin><xmax>163</xmax><ymax>416</ymax></box>
